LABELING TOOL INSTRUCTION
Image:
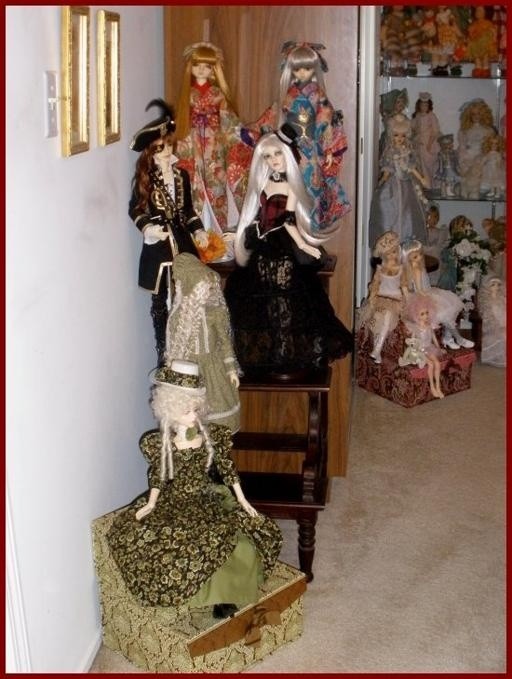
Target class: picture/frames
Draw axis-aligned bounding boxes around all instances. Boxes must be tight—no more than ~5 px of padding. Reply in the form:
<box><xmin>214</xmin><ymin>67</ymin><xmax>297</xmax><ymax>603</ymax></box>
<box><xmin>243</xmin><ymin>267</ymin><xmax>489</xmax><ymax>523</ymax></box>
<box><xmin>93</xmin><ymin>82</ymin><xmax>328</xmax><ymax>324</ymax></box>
<box><xmin>60</xmin><ymin>6</ymin><xmax>121</xmax><ymax>158</ymax></box>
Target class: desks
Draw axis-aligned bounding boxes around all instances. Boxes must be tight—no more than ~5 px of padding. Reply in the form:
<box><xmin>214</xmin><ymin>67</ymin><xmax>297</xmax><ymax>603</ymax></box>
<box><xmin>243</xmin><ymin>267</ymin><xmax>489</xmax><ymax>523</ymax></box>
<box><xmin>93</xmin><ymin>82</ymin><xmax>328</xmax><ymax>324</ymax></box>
<box><xmin>211</xmin><ymin>365</ymin><xmax>333</xmax><ymax>584</ymax></box>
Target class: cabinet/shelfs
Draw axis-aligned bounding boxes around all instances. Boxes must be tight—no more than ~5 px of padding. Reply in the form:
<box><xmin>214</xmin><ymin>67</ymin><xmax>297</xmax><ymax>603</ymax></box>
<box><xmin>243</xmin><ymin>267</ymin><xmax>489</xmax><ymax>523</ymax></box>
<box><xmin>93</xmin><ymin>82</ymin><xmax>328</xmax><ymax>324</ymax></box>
<box><xmin>163</xmin><ymin>1</ymin><xmax>512</xmax><ymax>474</ymax></box>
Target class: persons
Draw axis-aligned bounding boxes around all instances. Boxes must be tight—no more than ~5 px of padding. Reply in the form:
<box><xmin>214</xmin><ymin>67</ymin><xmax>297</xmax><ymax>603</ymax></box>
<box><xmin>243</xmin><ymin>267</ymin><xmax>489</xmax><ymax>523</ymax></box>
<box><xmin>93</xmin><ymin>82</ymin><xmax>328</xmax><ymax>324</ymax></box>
<box><xmin>238</xmin><ymin>41</ymin><xmax>352</xmax><ymax>235</ymax></box>
<box><xmin>129</xmin><ymin>94</ymin><xmax>207</xmax><ymax>365</ymax></box>
<box><xmin>220</xmin><ymin>123</ymin><xmax>355</xmax><ymax>380</ymax></box>
<box><xmin>170</xmin><ymin>39</ymin><xmax>245</xmax><ymax>232</ymax></box>
<box><xmin>354</xmin><ymin>5</ymin><xmax>508</xmax><ymax>405</ymax></box>
<box><xmin>167</xmin><ymin>252</ymin><xmax>242</xmax><ymax>411</ymax></box>
<box><xmin>98</xmin><ymin>354</ymin><xmax>286</xmax><ymax>622</ymax></box>
